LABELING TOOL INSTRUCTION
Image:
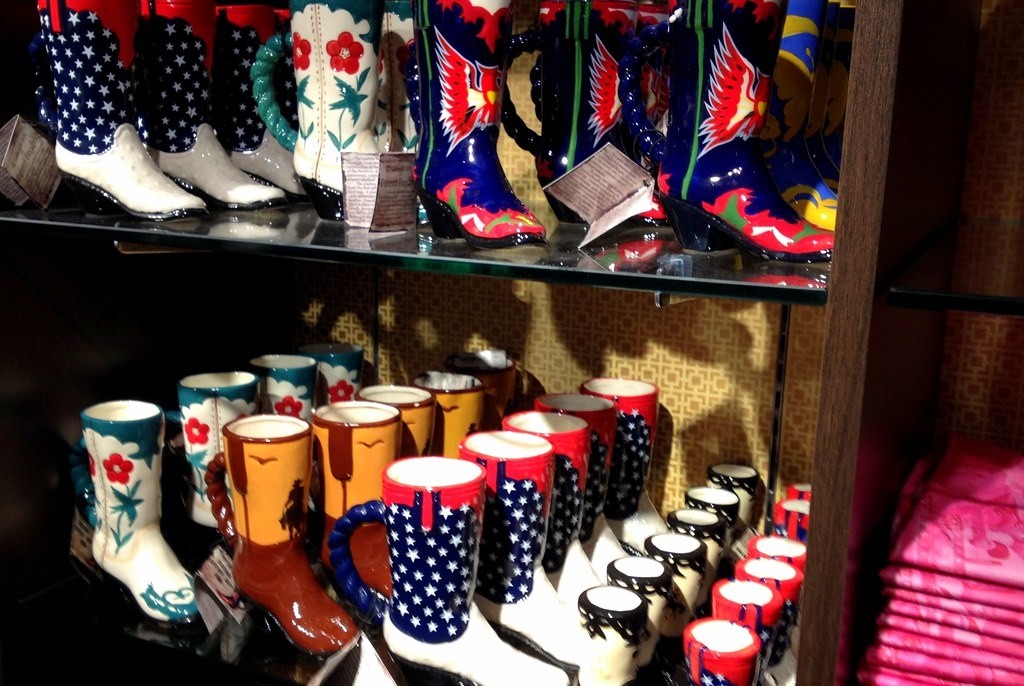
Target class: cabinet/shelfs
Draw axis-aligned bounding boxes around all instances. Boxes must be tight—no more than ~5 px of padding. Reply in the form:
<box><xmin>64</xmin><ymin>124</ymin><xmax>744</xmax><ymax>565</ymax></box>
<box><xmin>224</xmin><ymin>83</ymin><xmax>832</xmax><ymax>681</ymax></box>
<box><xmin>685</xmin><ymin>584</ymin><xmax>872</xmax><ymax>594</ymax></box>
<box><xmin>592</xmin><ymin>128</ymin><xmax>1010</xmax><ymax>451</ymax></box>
<box><xmin>0</xmin><ymin>0</ymin><xmax>982</xmax><ymax>686</ymax></box>
